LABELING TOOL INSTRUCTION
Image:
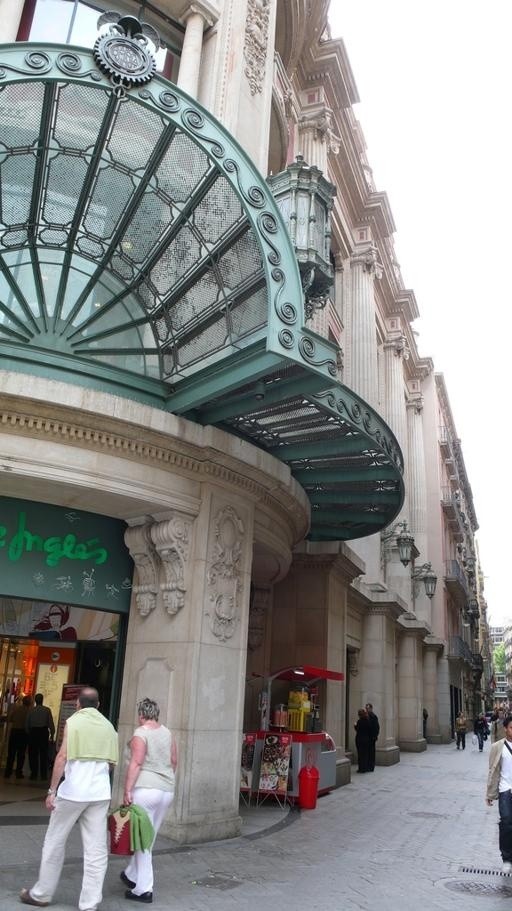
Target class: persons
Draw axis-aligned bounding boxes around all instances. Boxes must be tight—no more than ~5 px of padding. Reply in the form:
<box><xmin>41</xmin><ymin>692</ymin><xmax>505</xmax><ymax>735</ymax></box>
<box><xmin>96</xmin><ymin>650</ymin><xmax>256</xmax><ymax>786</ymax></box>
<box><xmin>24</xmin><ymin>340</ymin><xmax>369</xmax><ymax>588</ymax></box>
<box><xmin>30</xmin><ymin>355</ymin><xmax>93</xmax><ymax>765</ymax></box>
<box><xmin>482</xmin><ymin>700</ymin><xmax>512</xmax><ymax>745</ymax></box>
<box><xmin>365</xmin><ymin>703</ymin><xmax>380</xmax><ymax>773</ymax></box>
<box><xmin>474</xmin><ymin>712</ymin><xmax>488</xmax><ymax>752</ymax></box>
<box><xmin>454</xmin><ymin>712</ymin><xmax>468</xmax><ymax>750</ymax></box>
<box><xmin>120</xmin><ymin>697</ymin><xmax>179</xmax><ymax>904</ymax></box>
<box><xmin>422</xmin><ymin>708</ymin><xmax>429</xmax><ymax>738</ymax></box>
<box><xmin>6</xmin><ymin>696</ymin><xmax>32</xmax><ymax>779</ymax></box>
<box><xmin>24</xmin><ymin>692</ymin><xmax>56</xmax><ymax>781</ymax></box>
<box><xmin>354</xmin><ymin>708</ymin><xmax>373</xmax><ymax>773</ymax></box>
<box><xmin>18</xmin><ymin>684</ymin><xmax>120</xmax><ymax>911</ymax></box>
<box><xmin>486</xmin><ymin>714</ymin><xmax>512</xmax><ymax>873</ymax></box>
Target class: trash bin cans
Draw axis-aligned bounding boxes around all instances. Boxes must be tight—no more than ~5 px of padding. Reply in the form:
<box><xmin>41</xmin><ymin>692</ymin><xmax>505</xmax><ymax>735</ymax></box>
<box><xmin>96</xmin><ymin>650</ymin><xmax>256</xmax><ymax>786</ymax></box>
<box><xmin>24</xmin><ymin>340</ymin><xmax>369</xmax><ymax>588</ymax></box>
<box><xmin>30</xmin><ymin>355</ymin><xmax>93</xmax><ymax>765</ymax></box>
<box><xmin>298</xmin><ymin>766</ymin><xmax>320</xmax><ymax>809</ymax></box>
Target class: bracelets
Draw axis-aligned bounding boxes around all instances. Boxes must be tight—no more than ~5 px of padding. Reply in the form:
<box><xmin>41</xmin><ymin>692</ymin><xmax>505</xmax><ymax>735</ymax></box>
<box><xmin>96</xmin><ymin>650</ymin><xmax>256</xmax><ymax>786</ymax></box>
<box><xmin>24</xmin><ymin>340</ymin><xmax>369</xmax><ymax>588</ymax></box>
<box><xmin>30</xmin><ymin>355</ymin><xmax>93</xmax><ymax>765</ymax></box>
<box><xmin>46</xmin><ymin>788</ymin><xmax>54</xmax><ymax>794</ymax></box>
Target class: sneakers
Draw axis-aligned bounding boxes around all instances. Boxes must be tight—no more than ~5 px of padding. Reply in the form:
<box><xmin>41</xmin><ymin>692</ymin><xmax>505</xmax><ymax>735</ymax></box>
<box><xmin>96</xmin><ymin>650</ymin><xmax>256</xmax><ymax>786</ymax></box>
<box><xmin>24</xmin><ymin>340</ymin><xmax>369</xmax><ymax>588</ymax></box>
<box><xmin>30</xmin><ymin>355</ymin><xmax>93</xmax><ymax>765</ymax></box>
<box><xmin>19</xmin><ymin>886</ymin><xmax>48</xmax><ymax>908</ymax></box>
<box><xmin>502</xmin><ymin>861</ymin><xmax>512</xmax><ymax>874</ymax></box>
<box><xmin>356</xmin><ymin>766</ymin><xmax>375</xmax><ymax>773</ymax></box>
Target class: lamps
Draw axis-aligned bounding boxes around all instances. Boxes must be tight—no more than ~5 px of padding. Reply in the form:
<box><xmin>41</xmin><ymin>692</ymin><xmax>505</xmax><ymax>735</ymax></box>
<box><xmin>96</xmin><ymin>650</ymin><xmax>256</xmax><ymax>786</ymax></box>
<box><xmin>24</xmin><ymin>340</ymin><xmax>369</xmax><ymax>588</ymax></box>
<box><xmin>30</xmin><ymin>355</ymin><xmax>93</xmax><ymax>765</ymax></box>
<box><xmin>382</xmin><ymin>519</ymin><xmax>414</xmax><ymax>568</ymax></box>
<box><xmin>411</xmin><ymin>562</ymin><xmax>438</xmax><ymax>599</ymax></box>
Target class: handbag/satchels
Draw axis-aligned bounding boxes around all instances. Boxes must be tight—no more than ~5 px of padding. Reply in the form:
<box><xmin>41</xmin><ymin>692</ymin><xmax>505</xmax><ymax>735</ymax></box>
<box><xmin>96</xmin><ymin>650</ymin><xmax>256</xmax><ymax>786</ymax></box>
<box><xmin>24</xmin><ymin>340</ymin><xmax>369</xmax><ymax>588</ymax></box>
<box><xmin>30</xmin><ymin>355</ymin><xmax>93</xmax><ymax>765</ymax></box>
<box><xmin>108</xmin><ymin>808</ymin><xmax>135</xmax><ymax>856</ymax></box>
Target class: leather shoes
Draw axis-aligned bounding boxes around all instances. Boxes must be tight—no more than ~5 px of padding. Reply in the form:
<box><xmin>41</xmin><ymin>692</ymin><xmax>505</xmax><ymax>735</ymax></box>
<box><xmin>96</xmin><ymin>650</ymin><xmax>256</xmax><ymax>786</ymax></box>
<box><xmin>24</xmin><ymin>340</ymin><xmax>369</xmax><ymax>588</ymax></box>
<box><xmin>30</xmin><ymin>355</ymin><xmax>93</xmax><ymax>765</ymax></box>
<box><xmin>120</xmin><ymin>871</ymin><xmax>136</xmax><ymax>889</ymax></box>
<box><xmin>125</xmin><ymin>888</ymin><xmax>154</xmax><ymax>904</ymax></box>
